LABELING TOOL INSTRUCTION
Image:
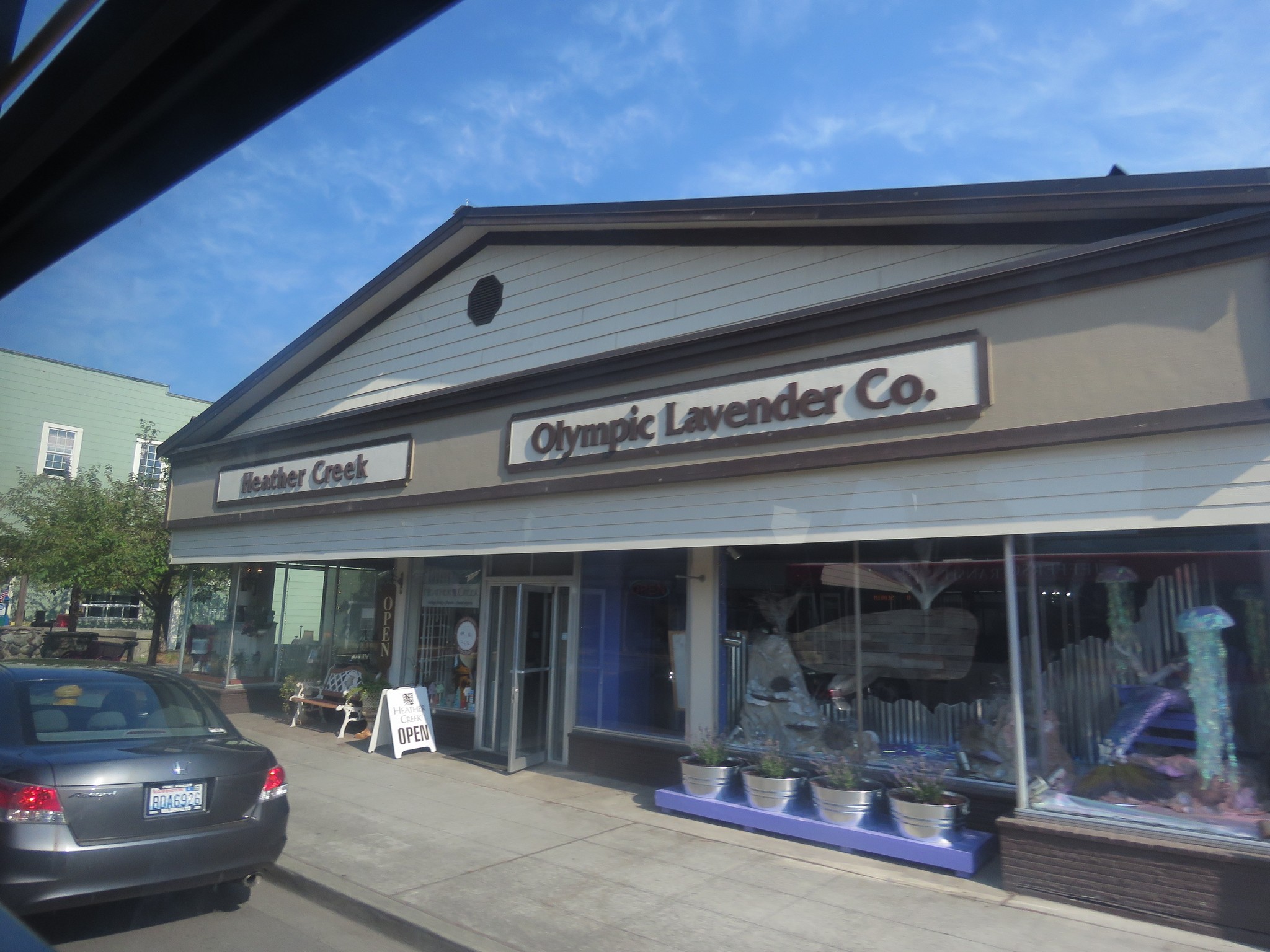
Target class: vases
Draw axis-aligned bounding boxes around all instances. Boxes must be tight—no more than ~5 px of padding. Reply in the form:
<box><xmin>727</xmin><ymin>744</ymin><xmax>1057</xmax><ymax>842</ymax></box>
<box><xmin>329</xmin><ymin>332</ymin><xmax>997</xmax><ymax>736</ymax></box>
<box><xmin>884</xmin><ymin>782</ymin><xmax>970</xmax><ymax>847</ymax></box>
<box><xmin>739</xmin><ymin>761</ymin><xmax>811</xmax><ymax>815</ymax></box>
<box><xmin>255</xmin><ymin>627</ymin><xmax>270</xmax><ymax>637</ymax></box>
<box><xmin>677</xmin><ymin>753</ymin><xmax>744</xmax><ymax>803</ymax></box>
<box><xmin>806</xmin><ymin>772</ymin><xmax>883</xmax><ymax>833</ymax></box>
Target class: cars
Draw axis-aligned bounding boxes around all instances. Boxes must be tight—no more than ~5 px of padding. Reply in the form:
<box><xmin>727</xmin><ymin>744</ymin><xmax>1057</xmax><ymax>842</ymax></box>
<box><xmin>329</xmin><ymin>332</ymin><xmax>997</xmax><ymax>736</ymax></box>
<box><xmin>1</xmin><ymin>660</ymin><xmax>290</xmax><ymax>920</ymax></box>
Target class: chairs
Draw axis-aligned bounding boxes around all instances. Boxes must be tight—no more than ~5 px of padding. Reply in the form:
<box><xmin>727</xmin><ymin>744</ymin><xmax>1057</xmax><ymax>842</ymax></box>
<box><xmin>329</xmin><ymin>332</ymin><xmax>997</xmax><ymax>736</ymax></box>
<box><xmin>0</xmin><ymin>687</ymin><xmax>206</xmax><ymax>740</ymax></box>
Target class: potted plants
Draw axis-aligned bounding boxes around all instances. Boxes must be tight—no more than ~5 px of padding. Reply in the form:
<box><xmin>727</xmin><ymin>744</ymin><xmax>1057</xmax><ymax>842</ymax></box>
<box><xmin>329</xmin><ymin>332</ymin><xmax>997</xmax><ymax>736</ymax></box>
<box><xmin>278</xmin><ymin>672</ymin><xmax>312</xmax><ymax>727</ymax></box>
<box><xmin>340</xmin><ymin>669</ymin><xmax>393</xmax><ymax>741</ymax></box>
<box><xmin>221</xmin><ymin>651</ymin><xmax>246</xmax><ymax>681</ymax></box>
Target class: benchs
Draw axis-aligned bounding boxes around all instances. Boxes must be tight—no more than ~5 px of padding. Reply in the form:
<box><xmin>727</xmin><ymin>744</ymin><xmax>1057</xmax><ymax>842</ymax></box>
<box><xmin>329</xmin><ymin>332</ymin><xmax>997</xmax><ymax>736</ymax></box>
<box><xmin>58</xmin><ymin>638</ymin><xmax>139</xmax><ymax>665</ymax></box>
<box><xmin>286</xmin><ymin>664</ymin><xmax>383</xmax><ymax>741</ymax></box>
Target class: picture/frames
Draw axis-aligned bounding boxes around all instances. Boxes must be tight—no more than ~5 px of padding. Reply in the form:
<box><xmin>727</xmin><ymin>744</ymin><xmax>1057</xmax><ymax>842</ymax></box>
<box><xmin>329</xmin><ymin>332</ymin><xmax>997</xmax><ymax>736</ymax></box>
<box><xmin>666</xmin><ymin>628</ymin><xmax>690</xmax><ymax>715</ymax></box>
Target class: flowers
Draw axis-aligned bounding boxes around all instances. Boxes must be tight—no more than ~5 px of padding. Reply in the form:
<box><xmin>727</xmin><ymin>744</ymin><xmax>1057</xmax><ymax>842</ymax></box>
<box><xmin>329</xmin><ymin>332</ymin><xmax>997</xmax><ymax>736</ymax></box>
<box><xmin>881</xmin><ymin>751</ymin><xmax>956</xmax><ymax>806</ymax></box>
<box><xmin>805</xmin><ymin>741</ymin><xmax>880</xmax><ymax>791</ymax></box>
<box><xmin>680</xmin><ymin>720</ymin><xmax>745</xmax><ymax>766</ymax></box>
<box><xmin>747</xmin><ymin>728</ymin><xmax>799</xmax><ymax>779</ymax></box>
<box><xmin>240</xmin><ymin>616</ymin><xmax>274</xmax><ymax>639</ymax></box>
